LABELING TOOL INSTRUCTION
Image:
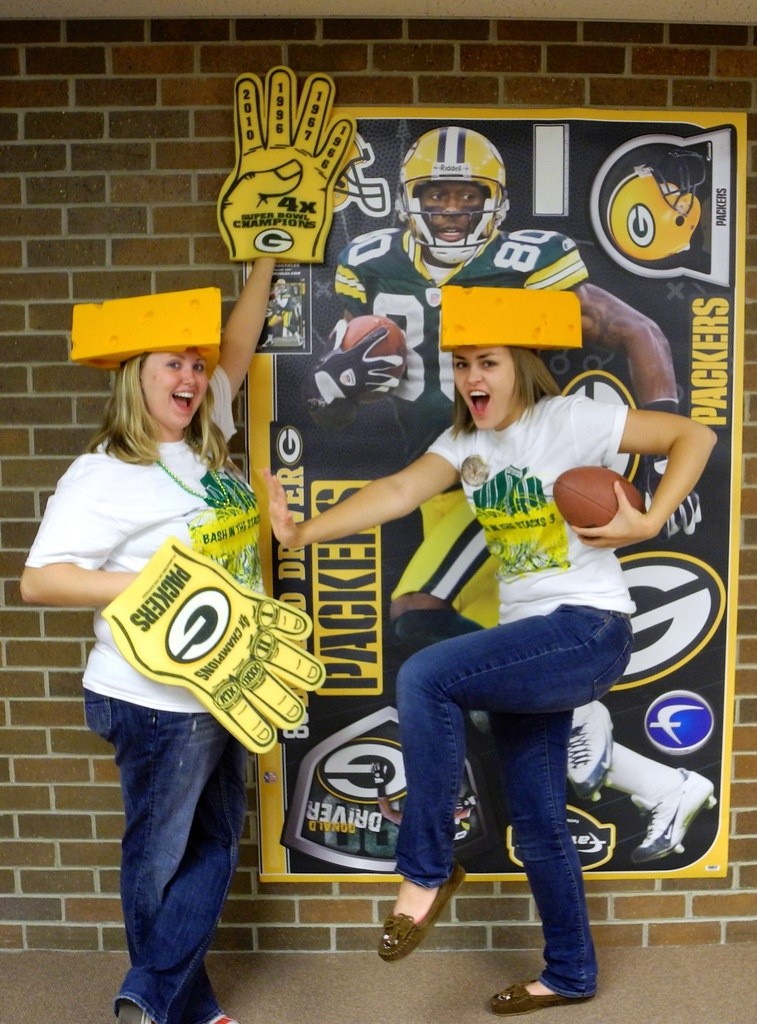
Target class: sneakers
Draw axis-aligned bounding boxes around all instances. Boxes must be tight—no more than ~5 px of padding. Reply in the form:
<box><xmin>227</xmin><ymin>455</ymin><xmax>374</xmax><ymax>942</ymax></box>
<box><xmin>566</xmin><ymin>700</ymin><xmax>613</xmax><ymax>800</ymax></box>
<box><xmin>631</xmin><ymin>767</ymin><xmax>717</xmax><ymax>869</ymax></box>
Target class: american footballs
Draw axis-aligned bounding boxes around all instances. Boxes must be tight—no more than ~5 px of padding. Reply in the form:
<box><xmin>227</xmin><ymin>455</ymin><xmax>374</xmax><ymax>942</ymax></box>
<box><xmin>342</xmin><ymin>313</ymin><xmax>409</xmax><ymax>402</ymax></box>
<box><xmin>553</xmin><ymin>466</ymin><xmax>647</xmax><ymax>540</ymax></box>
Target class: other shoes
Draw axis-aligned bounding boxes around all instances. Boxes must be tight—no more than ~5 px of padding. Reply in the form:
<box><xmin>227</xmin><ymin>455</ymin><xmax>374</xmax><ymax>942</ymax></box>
<box><xmin>297</xmin><ymin>337</ymin><xmax>305</xmax><ymax>347</ymax></box>
<box><xmin>116</xmin><ymin>999</ymin><xmax>242</xmax><ymax>1024</ymax></box>
<box><xmin>490</xmin><ymin>979</ymin><xmax>596</xmax><ymax>1017</ymax></box>
<box><xmin>378</xmin><ymin>860</ymin><xmax>466</xmax><ymax>961</ymax></box>
<box><xmin>262</xmin><ymin>341</ymin><xmax>273</xmax><ymax>347</ymax></box>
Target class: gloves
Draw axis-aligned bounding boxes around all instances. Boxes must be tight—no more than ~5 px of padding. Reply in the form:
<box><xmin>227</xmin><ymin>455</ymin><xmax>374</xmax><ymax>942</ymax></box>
<box><xmin>304</xmin><ymin>318</ymin><xmax>403</xmax><ymax>412</ymax></box>
<box><xmin>637</xmin><ymin>399</ymin><xmax>702</xmax><ymax>541</ymax></box>
<box><xmin>371</xmin><ymin>762</ymin><xmax>388</xmax><ymax>784</ymax></box>
<box><xmin>455</xmin><ymin>796</ymin><xmax>476</xmax><ymax>815</ymax></box>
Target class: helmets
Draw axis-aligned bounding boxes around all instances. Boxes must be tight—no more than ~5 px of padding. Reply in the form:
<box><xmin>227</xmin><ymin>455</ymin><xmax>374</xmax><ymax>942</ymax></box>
<box><xmin>277</xmin><ymin>279</ymin><xmax>286</xmax><ymax>285</ymax></box>
<box><xmin>394</xmin><ymin>126</ymin><xmax>511</xmax><ymax>265</ymax></box>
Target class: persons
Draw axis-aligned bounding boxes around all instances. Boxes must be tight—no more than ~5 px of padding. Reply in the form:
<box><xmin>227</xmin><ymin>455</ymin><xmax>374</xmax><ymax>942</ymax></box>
<box><xmin>22</xmin><ymin>260</ymin><xmax>326</xmax><ymax>1024</ymax></box>
<box><xmin>301</xmin><ymin>125</ymin><xmax>715</xmax><ymax>862</ymax></box>
<box><xmin>254</xmin><ymin>285</ymin><xmax>719</xmax><ymax>1018</ymax></box>
